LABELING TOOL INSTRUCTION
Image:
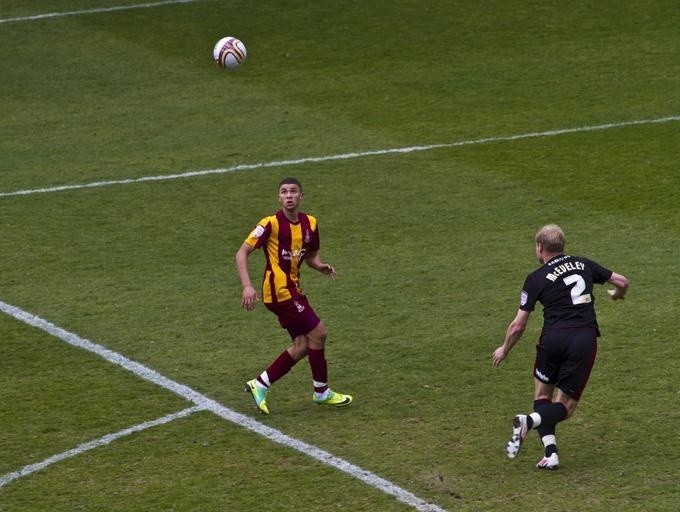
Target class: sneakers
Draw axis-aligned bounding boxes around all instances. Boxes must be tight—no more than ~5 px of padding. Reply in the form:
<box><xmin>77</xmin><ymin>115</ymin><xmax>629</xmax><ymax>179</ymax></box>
<box><xmin>506</xmin><ymin>413</ymin><xmax>529</xmax><ymax>460</ymax></box>
<box><xmin>534</xmin><ymin>452</ymin><xmax>560</xmax><ymax>470</ymax></box>
<box><xmin>311</xmin><ymin>391</ymin><xmax>352</xmax><ymax>406</ymax></box>
<box><xmin>245</xmin><ymin>379</ymin><xmax>271</xmax><ymax>416</ymax></box>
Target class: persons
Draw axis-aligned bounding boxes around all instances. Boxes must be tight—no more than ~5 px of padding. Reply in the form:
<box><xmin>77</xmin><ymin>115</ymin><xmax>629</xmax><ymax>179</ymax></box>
<box><xmin>491</xmin><ymin>223</ymin><xmax>630</xmax><ymax>470</ymax></box>
<box><xmin>234</xmin><ymin>176</ymin><xmax>355</xmax><ymax>416</ymax></box>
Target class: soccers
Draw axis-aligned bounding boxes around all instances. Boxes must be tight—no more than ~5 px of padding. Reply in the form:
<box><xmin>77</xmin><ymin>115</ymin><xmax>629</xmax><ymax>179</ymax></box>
<box><xmin>213</xmin><ymin>37</ymin><xmax>246</xmax><ymax>68</ymax></box>
